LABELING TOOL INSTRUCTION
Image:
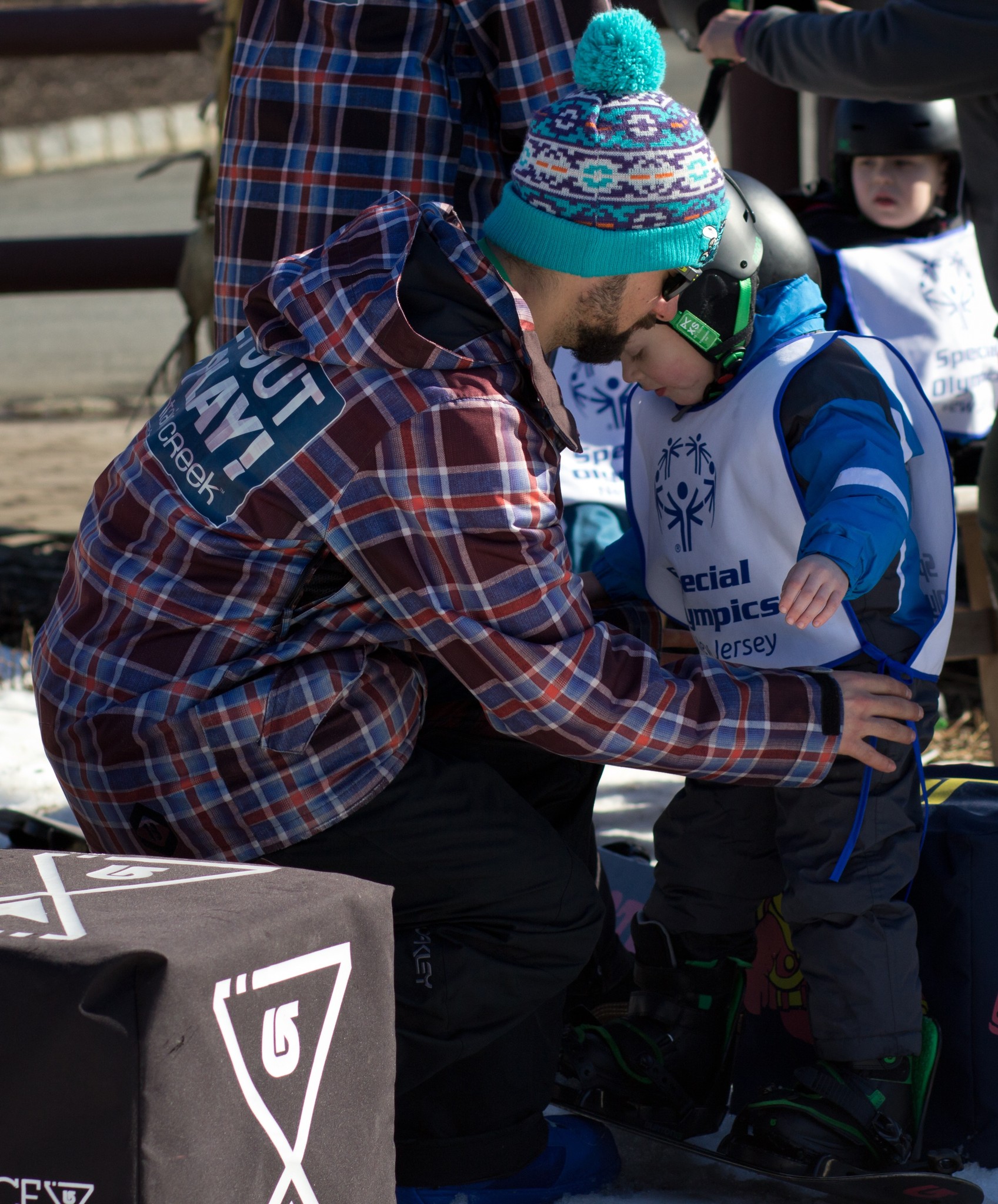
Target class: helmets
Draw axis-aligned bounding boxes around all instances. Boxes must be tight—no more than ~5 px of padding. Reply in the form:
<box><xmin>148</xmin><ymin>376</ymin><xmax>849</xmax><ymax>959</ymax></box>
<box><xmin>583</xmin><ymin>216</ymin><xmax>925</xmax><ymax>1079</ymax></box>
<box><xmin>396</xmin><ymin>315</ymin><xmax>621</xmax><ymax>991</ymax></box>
<box><xmin>831</xmin><ymin>97</ymin><xmax>963</xmax><ymax>218</ymax></box>
<box><xmin>707</xmin><ymin>168</ymin><xmax>823</xmax><ymax>295</ymax></box>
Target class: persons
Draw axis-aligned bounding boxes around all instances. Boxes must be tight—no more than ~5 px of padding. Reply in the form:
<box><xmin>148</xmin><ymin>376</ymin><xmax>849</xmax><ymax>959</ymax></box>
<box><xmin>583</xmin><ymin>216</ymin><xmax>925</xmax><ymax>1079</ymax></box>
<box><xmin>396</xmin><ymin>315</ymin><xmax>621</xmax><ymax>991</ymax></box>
<box><xmin>25</xmin><ymin>4</ymin><xmax>929</xmax><ymax>1118</ymax></box>
<box><xmin>220</xmin><ymin>0</ymin><xmax>998</xmax><ymax>1204</ymax></box>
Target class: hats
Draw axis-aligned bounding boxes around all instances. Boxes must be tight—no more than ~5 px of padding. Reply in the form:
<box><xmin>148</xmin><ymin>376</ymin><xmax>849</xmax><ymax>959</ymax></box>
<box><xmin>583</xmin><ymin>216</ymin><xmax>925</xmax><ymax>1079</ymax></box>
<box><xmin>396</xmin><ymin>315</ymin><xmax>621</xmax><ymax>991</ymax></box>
<box><xmin>478</xmin><ymin>6</ymin><xmax>732</xmax><ymax>278</ymax></box>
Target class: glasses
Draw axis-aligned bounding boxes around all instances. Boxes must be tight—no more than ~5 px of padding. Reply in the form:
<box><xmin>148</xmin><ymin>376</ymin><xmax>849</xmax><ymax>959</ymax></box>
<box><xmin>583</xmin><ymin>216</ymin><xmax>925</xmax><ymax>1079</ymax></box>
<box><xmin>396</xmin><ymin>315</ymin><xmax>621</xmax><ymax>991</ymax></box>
<box><xmin>661</xmin><ymin>265</ymin><xmax>702</xmax><ymax>303</ymax></box>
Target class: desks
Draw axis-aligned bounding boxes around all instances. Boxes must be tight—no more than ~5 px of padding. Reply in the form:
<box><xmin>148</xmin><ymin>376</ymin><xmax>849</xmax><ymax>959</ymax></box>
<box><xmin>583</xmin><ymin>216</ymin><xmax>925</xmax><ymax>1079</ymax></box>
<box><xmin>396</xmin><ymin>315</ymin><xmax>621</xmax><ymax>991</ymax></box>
<box><xmin>0</xmin><ymin>848</ymin><xmax>399</xmax><ymax>1204</ymax></box>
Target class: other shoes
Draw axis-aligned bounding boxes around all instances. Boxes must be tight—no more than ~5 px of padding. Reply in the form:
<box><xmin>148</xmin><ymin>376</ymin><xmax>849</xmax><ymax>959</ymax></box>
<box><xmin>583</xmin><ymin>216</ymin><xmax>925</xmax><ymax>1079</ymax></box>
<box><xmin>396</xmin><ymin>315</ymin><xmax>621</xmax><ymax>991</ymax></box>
<box><xmin>551</xmin><ymin>906</ymin><xmax>756</xmax><ymax>1141</ymax></box>
<box><xmin>396</xmin><ymin>1113</ymin><xmax>624</xmax><ymax>1204</ymax></box>
<box><xmin>739</xmin><ymin>1015</ymin><xmax>964</xmax><ymax>1177</ymax></box>
